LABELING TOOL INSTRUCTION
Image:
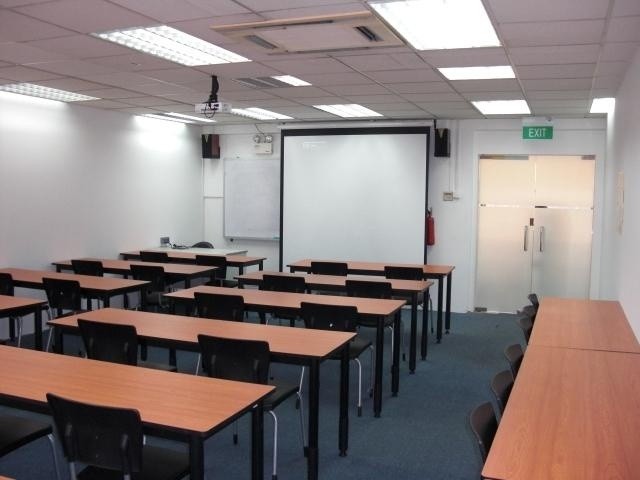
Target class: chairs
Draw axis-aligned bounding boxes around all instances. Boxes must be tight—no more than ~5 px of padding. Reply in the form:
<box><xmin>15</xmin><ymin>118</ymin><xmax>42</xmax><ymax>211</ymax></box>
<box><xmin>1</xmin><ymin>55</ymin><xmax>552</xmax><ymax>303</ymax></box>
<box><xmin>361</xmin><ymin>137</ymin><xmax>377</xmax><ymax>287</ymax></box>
<box><xmin>506</xmin><ymin>344</ymin><xmax>524</xmax><ymax>382</ymax></box>
<box><xmin>196</xmin><ymin>333</ymin><xmax>309</xmax><ymax>478</ymax></box>
<box><xmin>45</xmin><ymin>393</ymin><xmax>190</xmax><ymax>479</ymax></box>
<box><xmin>1</xmin><ymin>414</ymin><xmax>61</xmax><ymax>479</ymax></box>
<box><xmin>491</xmin><ymin>370</ymin><xmax>514</xmax><ymax>415</ymax></box>
<box><xmin>77</xmin><ymin>318</ymin><xmax>177</xmax><ymax>445</ymax></box>
<box><xmin>517</xmin><ymin>294</ymin><xmax>540</xmax><ymax>346</ymax></box>
<box><xmin>469</xmin><ymin>402</ymin><xmax>498</xmax><ymax>464</ymax></box>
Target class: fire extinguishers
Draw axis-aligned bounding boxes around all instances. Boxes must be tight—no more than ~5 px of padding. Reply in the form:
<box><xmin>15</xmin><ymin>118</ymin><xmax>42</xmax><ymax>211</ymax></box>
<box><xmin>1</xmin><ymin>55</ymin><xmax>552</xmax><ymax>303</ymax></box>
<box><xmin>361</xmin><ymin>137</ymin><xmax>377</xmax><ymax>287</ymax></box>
<box><xmin>426</xmin><ymin>207</ymin><xmax>434</xmax><ymax>245</ymax></box>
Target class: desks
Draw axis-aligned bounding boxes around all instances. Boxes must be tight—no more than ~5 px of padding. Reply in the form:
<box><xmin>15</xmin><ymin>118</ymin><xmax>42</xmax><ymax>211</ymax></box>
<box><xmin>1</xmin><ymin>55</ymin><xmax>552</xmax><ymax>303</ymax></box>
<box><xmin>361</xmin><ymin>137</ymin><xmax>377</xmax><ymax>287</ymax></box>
<box><xmin>480</xmin><ymin>344</ymin><xmax>640</xmax><ymax>479</ymax></box>
<box><xmin>164</xmin><ymin>259</ymin><xmax>455</xmax><ymax>419</ymax></box>
<box><xmin>46</xmin><ymin>308</ymin><xmax>359</xmax><ymax>479</ymax></box>
<box><xmin>1</xmin><ymin>345</ymin><xmax>276</xmax><ymax>480</ymax></box>
<box><xmin>527</xmin><ymin>296</ymin><xmax>640</xmax><ymax>353</ymax></box>
<box><xmin>1</xmin><ymin>241</ymin><xmax>266</xmax><ymax>366</ymax></box>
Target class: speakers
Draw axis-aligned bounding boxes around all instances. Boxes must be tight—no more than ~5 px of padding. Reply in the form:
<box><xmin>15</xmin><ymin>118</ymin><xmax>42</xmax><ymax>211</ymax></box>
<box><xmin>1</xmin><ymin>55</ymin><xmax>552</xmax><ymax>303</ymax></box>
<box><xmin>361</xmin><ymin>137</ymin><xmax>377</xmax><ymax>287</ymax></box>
<box><xmin>434</xmin><ymin>128</ymin><xmax>451</xmax><ymax>157</ymax></box>
<box><xmin>202</xmin><ymin>134</ymin><xmax>220</xmax><ymax>158</ymax></box>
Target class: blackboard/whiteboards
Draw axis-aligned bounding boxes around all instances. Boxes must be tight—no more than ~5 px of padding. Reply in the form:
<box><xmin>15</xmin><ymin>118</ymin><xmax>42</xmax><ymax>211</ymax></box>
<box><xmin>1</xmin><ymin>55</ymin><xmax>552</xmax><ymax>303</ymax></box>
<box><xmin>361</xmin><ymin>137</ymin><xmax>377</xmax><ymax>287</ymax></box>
<box><xmin>223</xmin><ymin>158</ymin><xmax>280</xmax><ymax>240</ymax></box>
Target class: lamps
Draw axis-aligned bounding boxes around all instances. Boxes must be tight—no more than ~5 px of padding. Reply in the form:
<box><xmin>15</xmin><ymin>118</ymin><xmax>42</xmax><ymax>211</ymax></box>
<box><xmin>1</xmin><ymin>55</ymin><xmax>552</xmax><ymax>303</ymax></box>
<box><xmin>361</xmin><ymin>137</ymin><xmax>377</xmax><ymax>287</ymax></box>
<box><xmin>250</xmin><ymin>134</ymin><xmax>274</xmax><ymax>155</ymax></box>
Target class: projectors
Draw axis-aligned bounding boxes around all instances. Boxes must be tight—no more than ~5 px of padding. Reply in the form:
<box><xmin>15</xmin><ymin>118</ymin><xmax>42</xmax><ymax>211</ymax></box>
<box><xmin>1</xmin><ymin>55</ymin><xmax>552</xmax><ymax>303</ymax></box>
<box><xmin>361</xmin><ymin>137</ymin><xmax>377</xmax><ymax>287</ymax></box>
<box><xmin>195</xmin><ymin>102</ymin><xmax>233</xmax><ymax>114</ymax></box>
